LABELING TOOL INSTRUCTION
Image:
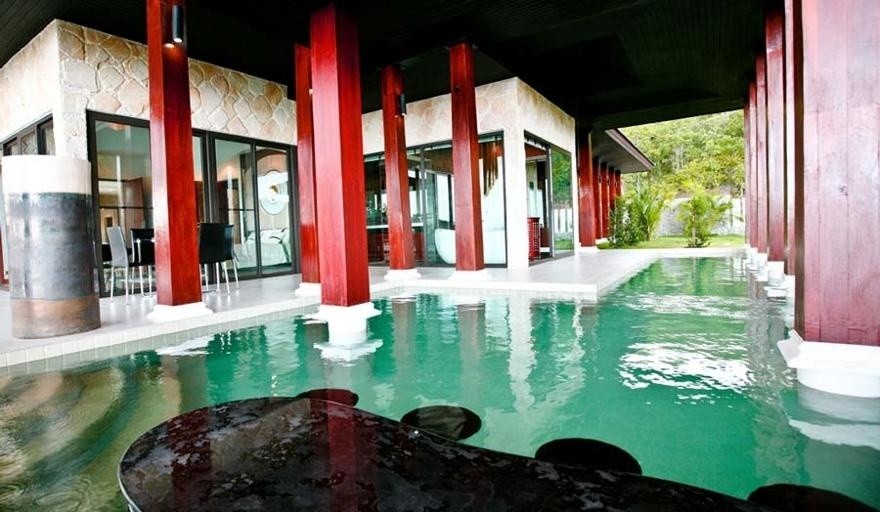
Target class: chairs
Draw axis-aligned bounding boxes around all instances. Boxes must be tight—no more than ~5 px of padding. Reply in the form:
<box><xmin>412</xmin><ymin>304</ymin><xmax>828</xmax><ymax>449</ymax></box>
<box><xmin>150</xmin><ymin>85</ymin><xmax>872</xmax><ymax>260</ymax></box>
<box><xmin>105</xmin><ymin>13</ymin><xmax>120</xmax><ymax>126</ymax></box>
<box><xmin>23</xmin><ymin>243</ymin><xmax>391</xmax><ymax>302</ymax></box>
<box><xmin>100</xmin><ymin>221</ymin><xmax>239</xmax><ymax>304</ymax></box>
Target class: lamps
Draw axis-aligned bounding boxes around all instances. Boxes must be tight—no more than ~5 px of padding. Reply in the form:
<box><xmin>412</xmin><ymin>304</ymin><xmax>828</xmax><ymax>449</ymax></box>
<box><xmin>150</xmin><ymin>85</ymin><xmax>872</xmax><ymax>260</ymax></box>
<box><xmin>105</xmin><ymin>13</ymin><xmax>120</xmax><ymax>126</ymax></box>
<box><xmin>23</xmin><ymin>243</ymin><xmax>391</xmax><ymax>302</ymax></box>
<box><xmin>163</xmin><ymin>17</ymin><xmax>174</xmax><ymax>48</ymax></box>
<box><xmin>171</xmin><ymin>3</ymin><xmax>185</xmax><ymax>43</ymax></box>
